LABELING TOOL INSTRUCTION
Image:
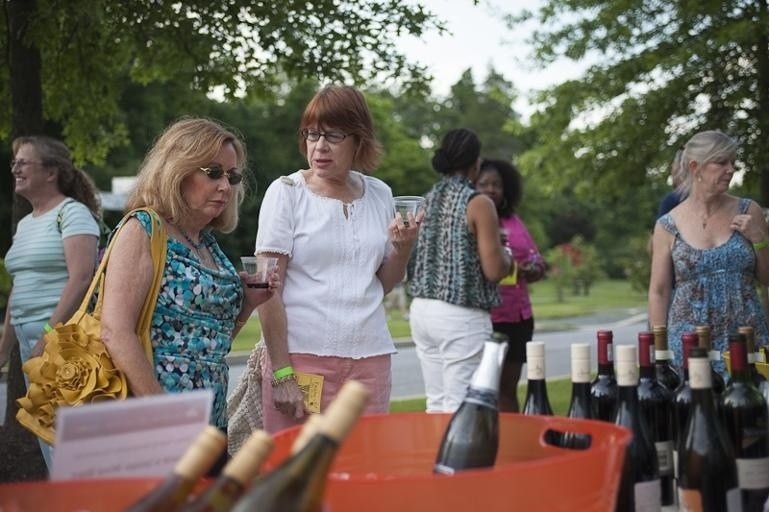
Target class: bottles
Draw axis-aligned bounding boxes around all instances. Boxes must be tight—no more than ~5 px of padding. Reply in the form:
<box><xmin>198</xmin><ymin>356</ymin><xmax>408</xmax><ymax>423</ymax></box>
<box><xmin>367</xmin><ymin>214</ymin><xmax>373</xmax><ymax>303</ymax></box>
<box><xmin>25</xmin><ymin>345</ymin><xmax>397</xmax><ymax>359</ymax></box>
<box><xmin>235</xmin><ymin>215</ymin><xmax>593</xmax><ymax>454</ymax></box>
<box><xmin>719</xmin><ymin>332</ymin><xmax>769</xmax><ymax>512</ymax></box>
<box><xmin>123</xmin><ymin>426</ymin><xmax>226</xmax><ymax>512</ymax></box>
<box><xmin>672</xmin><ymin>332</ymin><xmax>701</xmax><ymax>480</ymax></box>
<box><xmin>695</xmin><ymin>325</ymin><xmax>725</xmax><ymax>397</ymax></box>
<box><xmin>677</xmin><ymin>347</ymin><xmax>741</xmax><ymax>512</ymax></box>
<box><xmin>558</xmin><ymin>343</ymin><xmax>599</xmax><ymax>450</ymax></box>
<box><xmin>522</xmin><ymin>340</ymin><xmax>558</xmax><ymax>445</ymax></box>
<box><xmin>232</xmin><ymin>377</ymin><xmax>371</xmax><ymax>512</ymax></box>
<box><xmin>638</xmin><ymin>332</ymin><xmax>674</xmax><ymax>506</ymax></box>
<box><xmin>590</xmin><ymin>330</ymin><xmax>617</xmax><ymax>422</ymax></box>
<box><xmin>653</xmin><ymin>325</ymin><xmax>679</xmax><ymax>388</ymax></box>
<box><xmin>608</xmin><ymin>345</ymin><xmax>660</xmax><ymax>511</ymax></box>
<box><xmin>181</xmin><ymin>429</ymin><xmax>274</xmax><ymax>511</ymax></box>
<box><xmin>738</xmin><ymin>325</ymin><xmax>766</xmax><ymax>388</ymax></box>
<box><xmin>431</xmin><ymin>332</ymin><xmax>510</xmax><ymax>474</ymax></box>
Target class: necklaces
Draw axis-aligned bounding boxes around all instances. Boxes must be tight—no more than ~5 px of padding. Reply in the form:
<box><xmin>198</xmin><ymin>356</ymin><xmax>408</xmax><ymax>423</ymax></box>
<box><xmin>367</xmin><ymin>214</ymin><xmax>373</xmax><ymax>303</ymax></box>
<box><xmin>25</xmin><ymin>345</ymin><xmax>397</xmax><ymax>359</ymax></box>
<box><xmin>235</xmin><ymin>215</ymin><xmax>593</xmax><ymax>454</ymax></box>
<box><xmin>699</xmin><ymin>203</ymin><xmax>722</xmax><ymax>230</ymax></box>
<box><xmin>178</xmin><ymin>226</ymin><xmax>207</xmax><ymax>260</ymax></box>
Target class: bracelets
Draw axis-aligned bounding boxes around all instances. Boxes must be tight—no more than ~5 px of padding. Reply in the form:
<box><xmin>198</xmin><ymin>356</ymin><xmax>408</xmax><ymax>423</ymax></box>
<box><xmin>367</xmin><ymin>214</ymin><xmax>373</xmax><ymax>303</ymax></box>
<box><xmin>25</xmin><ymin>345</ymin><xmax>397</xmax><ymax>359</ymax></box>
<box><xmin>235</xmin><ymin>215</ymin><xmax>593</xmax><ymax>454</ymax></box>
<box><xmin>235</xmin><ymin>319</ymin><xmax>247</xmax><ymax>327</ymax></box>
<box><xmin>42</xmin><ymin>323</ymin><xmax>53</xmax><ymax>334</ymax></box>
<box><xmin>753</xmin><ymin>238</ymin><xmax>769</xmax><ymax>251</ymax></box>
<box><xmin>270</xmin><ymin>373</ymin><xmax>298</xmax><ymax>387</ymax></box>
<box><xmin>272</xmin><ymin>365</ymin><xmax>294</xmax><ymax>380</ymax></box>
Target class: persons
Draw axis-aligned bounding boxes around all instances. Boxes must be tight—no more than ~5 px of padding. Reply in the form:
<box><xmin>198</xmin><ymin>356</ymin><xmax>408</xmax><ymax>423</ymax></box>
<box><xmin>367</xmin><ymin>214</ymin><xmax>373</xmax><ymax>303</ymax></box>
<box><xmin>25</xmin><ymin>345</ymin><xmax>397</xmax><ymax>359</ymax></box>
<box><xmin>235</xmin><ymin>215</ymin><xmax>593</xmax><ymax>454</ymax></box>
<box><xmin>400</xmin><ymin>127</ymin><xmax>514</xmax><ymax>414</ymax></box>
<box><xmin>0</xmin><ymin>134</ymin><xmax>101</xmax><ymax>473</ymax></box>
<box><xmin>254</xmin><ymin>84</ymin><xmax>425</xmax><ymax>433</ymax></box>
<box><xmin>97</xmin><ymin>118</ymin><xmax>282</xmax><ymax>430</ymax></box>
<box><xmin>471</xmin><ymin>159</ymin><xmax>547</xmax><ymax>415</ymax></box>
<box><xmin>647</xmin><ymin>129</ymin><xmax>769</xmax><ymax>383</ymax></box>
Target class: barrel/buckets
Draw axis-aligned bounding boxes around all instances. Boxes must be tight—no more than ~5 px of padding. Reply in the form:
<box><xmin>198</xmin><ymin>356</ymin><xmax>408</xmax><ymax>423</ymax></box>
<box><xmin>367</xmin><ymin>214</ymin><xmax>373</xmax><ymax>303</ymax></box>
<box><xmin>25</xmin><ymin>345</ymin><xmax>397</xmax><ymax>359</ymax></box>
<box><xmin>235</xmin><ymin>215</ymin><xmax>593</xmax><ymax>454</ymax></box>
<box><xmin>258</xmin><ymin>411</ymin><xmax>633</xmax><ymax>512</ymax></box>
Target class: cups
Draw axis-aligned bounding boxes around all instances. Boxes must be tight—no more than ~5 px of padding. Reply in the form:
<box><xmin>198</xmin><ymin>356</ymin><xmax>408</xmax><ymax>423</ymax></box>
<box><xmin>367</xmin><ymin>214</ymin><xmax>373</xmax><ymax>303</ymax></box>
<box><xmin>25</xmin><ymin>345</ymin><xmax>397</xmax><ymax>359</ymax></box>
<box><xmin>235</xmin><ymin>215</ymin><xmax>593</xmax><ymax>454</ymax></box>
<box><xmin>393</xmin><ymin>197</ymin><xmax>425</xmax><ymax>225</ymax></box>
<box><xmin>240</xmin><ymin>257</ymin><xmax>278</xmax><ymax>288</ymax></box>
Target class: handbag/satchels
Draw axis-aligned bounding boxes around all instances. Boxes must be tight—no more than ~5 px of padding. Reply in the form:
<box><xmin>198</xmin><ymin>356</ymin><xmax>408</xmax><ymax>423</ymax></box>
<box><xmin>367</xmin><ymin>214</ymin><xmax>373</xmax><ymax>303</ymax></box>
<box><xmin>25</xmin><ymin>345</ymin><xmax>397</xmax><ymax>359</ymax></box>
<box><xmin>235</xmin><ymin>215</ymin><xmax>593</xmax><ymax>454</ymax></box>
<box><xmin>16</xmin><ymin>207</ymin><xmax>167</xmax><ymax>444</ymax></box>
<box><xmin>226</xmin><ymin>338</ymin><xmax>262</xmax><ymax>457</ymax></box>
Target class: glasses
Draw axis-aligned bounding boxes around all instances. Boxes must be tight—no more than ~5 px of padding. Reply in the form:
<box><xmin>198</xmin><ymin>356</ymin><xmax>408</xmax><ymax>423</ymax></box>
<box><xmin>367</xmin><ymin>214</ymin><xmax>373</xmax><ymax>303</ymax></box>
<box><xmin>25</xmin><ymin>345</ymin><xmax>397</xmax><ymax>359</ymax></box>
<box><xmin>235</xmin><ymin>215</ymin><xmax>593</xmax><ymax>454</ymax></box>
<box><xmin>199</xmin><ymin>166</ymin><xmax>243</xmax><ymax>185</ymax></box>
<box><xmin>9</xmin><ymin>157</ymin><xmax>41</xmax><ymax>172</ymax></box>
<box><xmin>301</xmin><ymin>127</ymin><xmax>353</xmax><ymax>144</ymax></box>
